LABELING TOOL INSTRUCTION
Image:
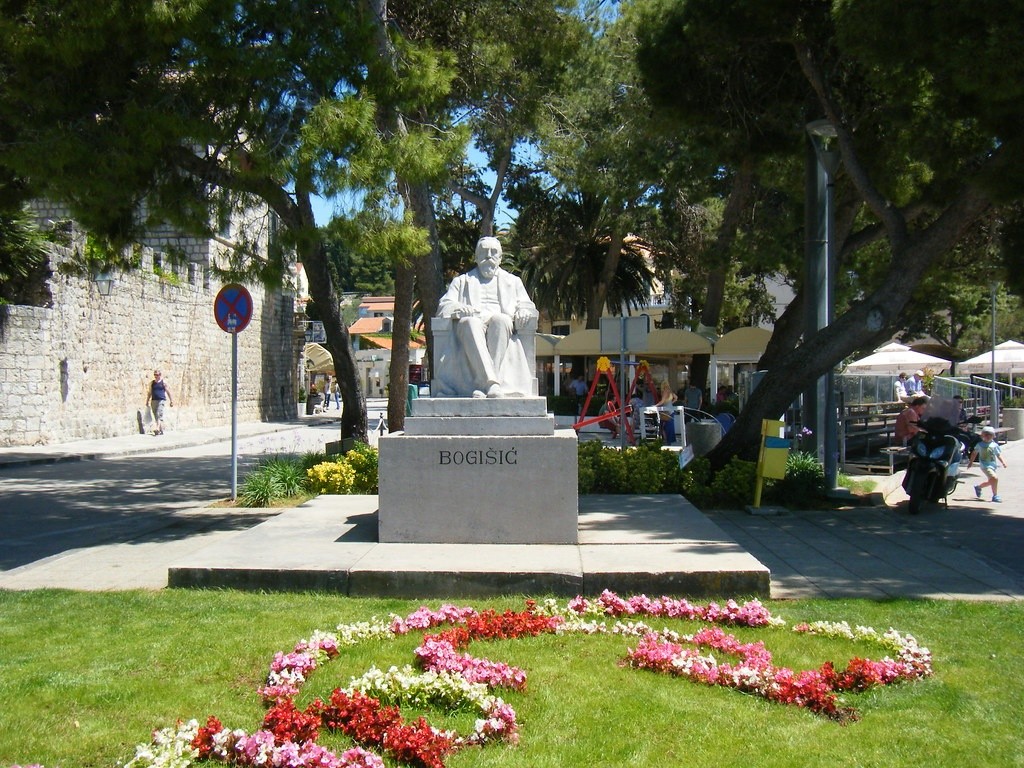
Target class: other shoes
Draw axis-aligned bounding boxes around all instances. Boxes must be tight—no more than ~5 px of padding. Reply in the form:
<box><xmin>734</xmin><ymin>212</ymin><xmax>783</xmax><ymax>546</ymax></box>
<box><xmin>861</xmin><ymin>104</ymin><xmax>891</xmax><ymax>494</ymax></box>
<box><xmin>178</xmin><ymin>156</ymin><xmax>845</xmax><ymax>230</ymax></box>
<box><xmin>158</xmin><ymin>430</ymin><xmax>164</xmax><ymax>435</ymax></box>
<box><xmin>153</xmin><ymin>429</ymin><xmax>159</xmax><ymax>435</ymax></box>
<box><xmin>992</xmin><ymin>495</ymin><xmax>1002</xmax><ymax>503</ymax></box>
<box><xmin>974</xmin><ymin>485</ymin><xmax>982</xmax><ymax>498</ymax></box>
<box><xmin>336</xmin><ymin>408</ymin><xmax>339</xmax><ymax>410</ymax></box>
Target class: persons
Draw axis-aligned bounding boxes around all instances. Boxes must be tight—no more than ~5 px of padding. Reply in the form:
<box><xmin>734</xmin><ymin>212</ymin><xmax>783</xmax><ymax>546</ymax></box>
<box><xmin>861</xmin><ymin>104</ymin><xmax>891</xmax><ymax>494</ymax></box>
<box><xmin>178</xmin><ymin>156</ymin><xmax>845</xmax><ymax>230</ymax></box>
<box><xmin>335</xmin><ymin>380</ymin><xmax>342</xmax><ymax>409</ymax></box>
<box><xmin>323</xmin><ymin>378</ymin><xmax>331</xmax><ymax>412</ymax></box>
<box><xmin>435</xmin><ymin>238</ymin><xmax>533</xmax><ymax>399</ymax></box>
<box><xmin>651</xmin><ymin>380</ymin><xmax>678</xmax><ymax>446</ymax></box>
<box><xmin>627</xmin><ymin>394</ymin><xmax>643</xmax><ymax>445</ymax></box>
<box><xmin>967</xmin><ymin>427</ymin><xmax>1007</xmax><ymax>503</ymax></box>
<box><xmin>905</xmin><ymin>371</ymin><xmax>930</xmax><ymax>398</ymax></box>
<box><xmin>894</xmin><ymin>373</ymin><xmax>919</xmax><ymax>402</ymax></box>
<box><xmin>944</xmin><ymin>395</ymin><xmax>983</xmax><ymax>462</ymax></box>
<box><xmin>684</xmin><ymin>382</ymin><xmax>702</xmax><ymax>424</ymax></box>
<box><xmin>569</xmin><ymin>375</ymin><xmax>589</xmax><ymax>415</ymax></box>
<box><xmin>146</xmin><ymin>370</ymin><xmax>173</xmax><ymax>434</ymax></box>
<box><xmin>718</xmin><ymin>386</ymin><xmax>727</xmax><ymax>402</ymax></box>
<box><xmin>895</xmin><ymin>396</ymin><xmax>928</xmax><ymax>441</ymax></box>
<box><xmin>598</xmin><ymin>395</ymin><xmax>638</xmax><ymax>442</ymax></box>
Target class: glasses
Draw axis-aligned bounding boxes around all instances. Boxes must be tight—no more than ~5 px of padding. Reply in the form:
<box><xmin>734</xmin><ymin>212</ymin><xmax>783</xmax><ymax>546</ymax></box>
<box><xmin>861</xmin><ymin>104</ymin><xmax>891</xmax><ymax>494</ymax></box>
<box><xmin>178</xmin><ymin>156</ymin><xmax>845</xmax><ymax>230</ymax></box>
<box><xmin>959</xmin><ymin>401</ymin><xmax>962</xmax><ymax>403</ymax></box>
<box><xmin>902</xmin><ymin>377</ymin><xmax>907</xmax><ymax>378</ymax></box>
<box><xmin>154</xmin><ymin>374</ymin><xmax>159</xmax><ymax>376</ymax></box>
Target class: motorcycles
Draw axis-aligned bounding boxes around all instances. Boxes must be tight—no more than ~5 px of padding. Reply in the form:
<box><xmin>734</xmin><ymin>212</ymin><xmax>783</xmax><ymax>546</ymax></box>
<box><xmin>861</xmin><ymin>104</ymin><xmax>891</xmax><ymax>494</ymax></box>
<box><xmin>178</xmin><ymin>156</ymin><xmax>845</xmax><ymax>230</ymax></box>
<box><xmin>898</xmin><ymin>391</ymin><xmax>984</xmax><ymax>515</ymax></box>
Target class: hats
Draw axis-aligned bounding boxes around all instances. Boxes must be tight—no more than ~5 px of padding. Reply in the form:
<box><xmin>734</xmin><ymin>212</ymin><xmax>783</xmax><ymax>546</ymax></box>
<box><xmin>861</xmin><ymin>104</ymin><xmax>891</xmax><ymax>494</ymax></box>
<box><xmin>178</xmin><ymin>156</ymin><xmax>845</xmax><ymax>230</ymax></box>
<box><xmin>914</xmin><ymin>370</ymin><xmax>924</xmax><ymax>377</ymax></box>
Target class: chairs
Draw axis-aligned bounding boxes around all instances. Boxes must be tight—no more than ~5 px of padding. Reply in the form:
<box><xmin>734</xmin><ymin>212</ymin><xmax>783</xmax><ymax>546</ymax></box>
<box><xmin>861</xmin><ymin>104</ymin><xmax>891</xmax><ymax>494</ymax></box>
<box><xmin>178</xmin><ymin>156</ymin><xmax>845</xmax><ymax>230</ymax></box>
<box><xmin>430</xmin><ymin>316</ymin><xmax>540</xmax><ymax>397</ymax></box>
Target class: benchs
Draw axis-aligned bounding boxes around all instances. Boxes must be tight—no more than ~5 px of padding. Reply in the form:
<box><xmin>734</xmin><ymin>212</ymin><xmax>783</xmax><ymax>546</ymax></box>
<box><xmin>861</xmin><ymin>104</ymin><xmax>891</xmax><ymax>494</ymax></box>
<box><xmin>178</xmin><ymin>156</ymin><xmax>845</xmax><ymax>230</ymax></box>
<box><xmin>845</xmin><ymin>398</ymin><xmax>1014</xmax><ymax>470</ymax></box>
